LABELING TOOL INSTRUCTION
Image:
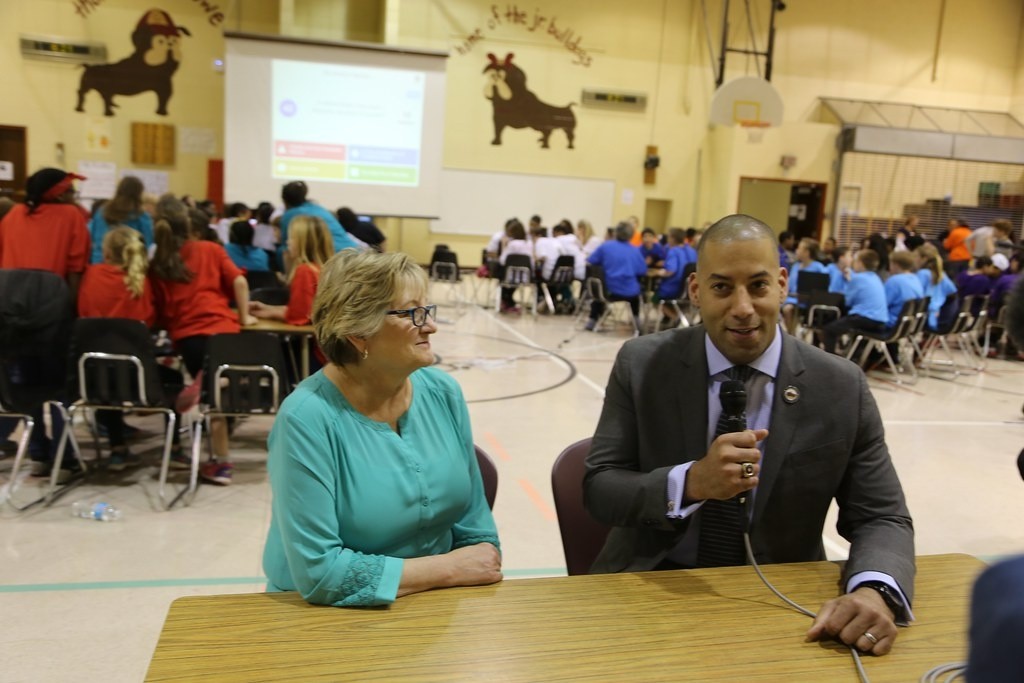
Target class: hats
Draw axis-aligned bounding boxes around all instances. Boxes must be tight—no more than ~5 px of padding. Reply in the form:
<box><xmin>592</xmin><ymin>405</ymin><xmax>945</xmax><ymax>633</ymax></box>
<box><xmin>992</xmin><ymin>253</ymin><xmax>1008</xmax><ymax>270</ymax></box>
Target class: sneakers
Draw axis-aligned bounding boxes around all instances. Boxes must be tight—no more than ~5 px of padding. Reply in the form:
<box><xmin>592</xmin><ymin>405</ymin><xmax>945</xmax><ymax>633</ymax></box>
<box><xmin>201</xmin><ymin>457</ymin><xmax>231</xmax><ymax>484</ymax></box>
<box><xmin>160</xmin><ymin>448</ymin><xmax>191</xmax><ymax>468</ymax></box>
<box><xmin>108</xmin><ymin>451</ymin><xmax>138</xmax><ymax>470</ymax></box>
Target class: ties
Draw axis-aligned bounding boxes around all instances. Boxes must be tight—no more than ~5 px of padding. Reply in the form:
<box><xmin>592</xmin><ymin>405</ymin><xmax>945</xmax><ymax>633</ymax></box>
<box><xmin>697</xmin><ymin>364</ymin><xmax>753</xmax><ymax>568</ymax></box>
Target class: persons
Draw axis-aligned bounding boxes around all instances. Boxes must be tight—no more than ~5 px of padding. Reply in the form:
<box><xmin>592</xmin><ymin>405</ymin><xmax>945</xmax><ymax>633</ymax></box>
<box><xmin>967</xmin><ymin>553</ymin><xmax>1024</xmax><ymax>683</ymax></box>
<box><xmin>581</xmin><ymin>212</ymin><xmax>916</xmax><ymax>656</ymax></box>
<box><xmin>0</xmin><ymin>166</ymin><xmax>385</xmax><ymax>486</ymax></box>
<box><xmin>261</xmin><ymin>247</ymin><xmax>503</xmax><ymax>608</ymax></box>
<box><xmin>481</xmin><ymin>215</ymin><xmax>1024</xmax><ymax>374</ymax></box>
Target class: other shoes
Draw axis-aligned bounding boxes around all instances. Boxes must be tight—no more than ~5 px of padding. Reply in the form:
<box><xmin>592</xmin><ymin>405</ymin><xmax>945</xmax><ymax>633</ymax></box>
<box><xmin>585</xmin><ymin>318</ymin><xmax>596</xmax><ymax>333</ymax></box>
<box><xmin>29</xmin><ymin>459</ymin><xmax>57</xmax><ymax>476</ymax></box>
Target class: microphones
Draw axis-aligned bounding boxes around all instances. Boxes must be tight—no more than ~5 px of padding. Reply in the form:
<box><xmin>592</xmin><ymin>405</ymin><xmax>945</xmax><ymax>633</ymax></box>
<box><xmin>720</xmin><ymin>379</ymin><xmax>748</xmax><ymax>506</ymax></box>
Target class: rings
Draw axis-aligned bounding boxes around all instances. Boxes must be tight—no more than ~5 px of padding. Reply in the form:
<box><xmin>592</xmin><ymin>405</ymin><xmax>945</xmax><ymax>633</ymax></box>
<box><xmin>742</xmin><ymin>462</ymin><xmax>755</xmax><ymax>478</ymax></box>
<box><xmin>863</xmin><ymin>632</ymin><xmax>877</xmax><ymax>643</ymax></box>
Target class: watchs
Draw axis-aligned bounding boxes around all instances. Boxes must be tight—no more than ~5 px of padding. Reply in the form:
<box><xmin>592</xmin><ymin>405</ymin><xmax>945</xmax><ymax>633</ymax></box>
<box><xmin>856</xmin><ymin>580</ymin><xmax>905</xmax><ymax>620</ymax></box>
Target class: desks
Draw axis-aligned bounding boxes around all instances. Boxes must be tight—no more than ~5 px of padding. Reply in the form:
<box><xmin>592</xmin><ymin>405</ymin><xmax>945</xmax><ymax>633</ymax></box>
<box><xmin>141</xmin><ymin>554</ymin><xmax>991</xmax><ymax>683</ymax></box>
<box><xmin>230</xmin><ymin>307</ymin><xmax>316</xmax><ymax>387</ymax></box>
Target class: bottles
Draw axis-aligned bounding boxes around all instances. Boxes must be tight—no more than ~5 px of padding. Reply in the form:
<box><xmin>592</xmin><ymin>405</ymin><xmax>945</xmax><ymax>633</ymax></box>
<box><xmin>71</xmin><ymin>500</ymin><xmax>122</xmax><ymax>522</ymax></box>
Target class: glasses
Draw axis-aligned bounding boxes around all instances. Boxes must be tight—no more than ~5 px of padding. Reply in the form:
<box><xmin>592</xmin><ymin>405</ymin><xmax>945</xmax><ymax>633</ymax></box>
<box><xmin>386</xmin><ymin>303</ymin><xmax>435</xmax><ymax>327</ymax></box>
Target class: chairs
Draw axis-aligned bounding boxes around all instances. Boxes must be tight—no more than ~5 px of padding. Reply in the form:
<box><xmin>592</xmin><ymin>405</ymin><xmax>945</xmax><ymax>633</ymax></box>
<box><xmin>1</xmin><ymin>246</ymin><xmax>300</xmax><ymax>510</ymax></box>
<box><xmin>429</xmin><ymin>246</ymin><xmax>1024</xmax><ymax>387</ymax></box>
